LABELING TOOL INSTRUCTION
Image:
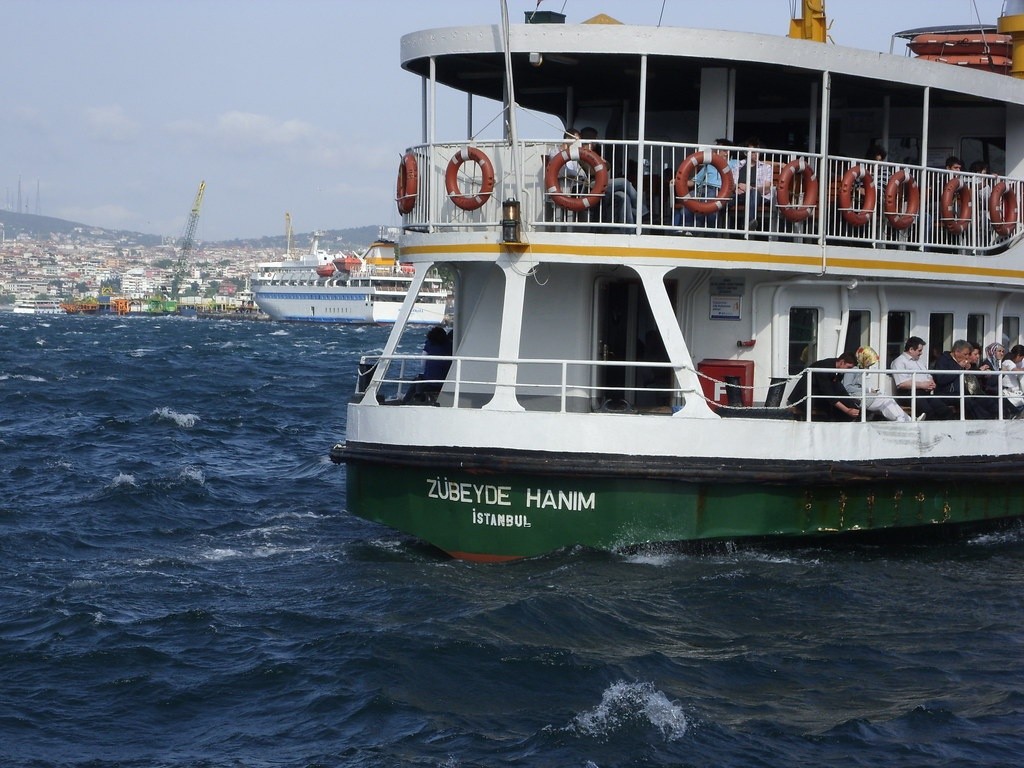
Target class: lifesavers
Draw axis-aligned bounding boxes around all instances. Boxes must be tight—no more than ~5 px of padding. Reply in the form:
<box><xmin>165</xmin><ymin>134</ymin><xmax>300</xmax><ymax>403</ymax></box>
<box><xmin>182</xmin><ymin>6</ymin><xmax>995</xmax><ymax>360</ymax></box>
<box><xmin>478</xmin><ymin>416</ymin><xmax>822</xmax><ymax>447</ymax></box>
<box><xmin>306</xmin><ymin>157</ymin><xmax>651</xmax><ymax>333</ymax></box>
<box><xmin>445</xmin><ymin>147</ymin><xmax>495</xmax><ymax>211</ymax></box>
<box><xmin>676</xmin><ymin>150</ymin><xmax>734</xmax><ymax>214</ymax></box>
<box><xmin>545</xmin><ymin>146</ymin><xmax>608</xmax><ymax>211</ymax></box>
<box><xmin>990</xmin><ymin>181</ymin><xmax>1017</xmax><ymax>233</ymax></box>
<box><xmin>942</xmin><ymin>178</ymin><xmax>972</xmax><ymax>235</ymax></box>
<box><xmin>907</xmin><ymin>33</ymin><xmax>1013</xmax><ymax>72</ymax></box>
<box><xmin>777</xmin><ymin>159</ymin><xmax>818</xmax><ymax>221</ymax></box>
<box><xmin>885</xmin><ymin>170</ymin><xmax>920</xmax><ymax>229</ymax></box>
<box><xmin>395</xmin><ymin>153</ymin><xmax>417</xmax><ymax>213</ymax></box>
<box><xmin>841</xmin><ymin>165</ymin><xmax>875</xmax><ymax>226</ymax></box>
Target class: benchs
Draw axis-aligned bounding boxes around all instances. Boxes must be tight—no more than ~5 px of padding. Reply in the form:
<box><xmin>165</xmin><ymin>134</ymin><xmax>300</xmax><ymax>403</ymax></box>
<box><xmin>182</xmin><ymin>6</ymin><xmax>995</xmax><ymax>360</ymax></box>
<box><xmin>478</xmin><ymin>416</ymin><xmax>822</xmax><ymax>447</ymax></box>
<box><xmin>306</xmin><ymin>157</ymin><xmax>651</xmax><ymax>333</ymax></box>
<box><xmin>669</xmin><ymin>160</ymin><xmax>856</xmax><ymax>242</ymax></box>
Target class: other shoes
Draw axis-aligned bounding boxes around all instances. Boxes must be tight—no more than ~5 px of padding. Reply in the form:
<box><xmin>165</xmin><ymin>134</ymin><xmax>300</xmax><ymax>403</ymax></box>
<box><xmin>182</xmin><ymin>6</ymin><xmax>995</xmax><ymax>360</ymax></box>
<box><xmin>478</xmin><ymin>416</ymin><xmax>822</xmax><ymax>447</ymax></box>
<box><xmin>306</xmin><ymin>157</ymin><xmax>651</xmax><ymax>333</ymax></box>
<box><xmin>917</xmin><ymin>413</ymin><xmax>926</xmax><ymax>421</ymax></box>
<box><xmin>1015</xmin><ymin>405</ymin><xmax>1024</xmax><ymax>413</ymax></box>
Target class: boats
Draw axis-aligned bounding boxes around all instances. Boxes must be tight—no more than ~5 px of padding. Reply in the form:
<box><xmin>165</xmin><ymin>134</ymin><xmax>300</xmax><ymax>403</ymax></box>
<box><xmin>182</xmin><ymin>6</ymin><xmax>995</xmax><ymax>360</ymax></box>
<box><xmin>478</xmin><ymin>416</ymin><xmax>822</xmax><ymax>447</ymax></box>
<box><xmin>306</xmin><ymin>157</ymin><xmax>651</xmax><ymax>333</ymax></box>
<box><xmin>332</xmin><ymin>256</ymin><xmax>361</xmax><ymax>273</ymax></box>
<box><xmin>910</xmin><ymin>52</ymin><xmax>1013</xmax><ymax>76</ymax></box>
<box><xmin>905</xmin><ymin>32</ymin><xmax>1014</xmax><ymax>57</ymax></box>
<box><xmin>246</xmin><ymin>224</ymin><xmax>449</xmax><ymax>325</ymax></box>
<box><xmin>326</xmin><ymin>0</ymin><xmax>1024</xmax><ymax>562</ymax></box>
<box><xmin>12</xmin><ymin>297</ymin><xmax>66</xmax><ymax>313</ymax></box>
<box><xmin>315</xmin><ymin>262</ymin><xmax>334</xmax><ymax>276</ymax></box>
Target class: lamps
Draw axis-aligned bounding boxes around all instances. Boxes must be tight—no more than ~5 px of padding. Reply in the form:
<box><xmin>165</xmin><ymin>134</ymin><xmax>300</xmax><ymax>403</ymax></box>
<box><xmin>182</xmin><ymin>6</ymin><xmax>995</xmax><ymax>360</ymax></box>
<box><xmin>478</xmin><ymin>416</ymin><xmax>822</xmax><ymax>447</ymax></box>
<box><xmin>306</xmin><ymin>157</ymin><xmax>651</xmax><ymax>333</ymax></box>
<box><xmin>499</xmin><ymin>197</ymin><xmax>521</xmax><ymax>242</ymax></box>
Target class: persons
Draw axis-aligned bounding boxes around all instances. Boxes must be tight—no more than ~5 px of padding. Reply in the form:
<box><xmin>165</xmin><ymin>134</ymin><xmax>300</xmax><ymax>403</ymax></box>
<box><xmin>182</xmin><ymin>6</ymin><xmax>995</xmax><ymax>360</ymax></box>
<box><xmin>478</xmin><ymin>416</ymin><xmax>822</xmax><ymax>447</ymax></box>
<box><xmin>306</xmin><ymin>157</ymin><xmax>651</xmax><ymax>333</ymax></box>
<box><xmin>890</xmin><ymin>336</ymin><xmax>954</xmax><ymax>419</ymax></box>
<box><xmin>553</xmin><ymin>128</ymin><xmax>649</xmax><ymax>232</ymax></box>
<box><xmin>842</xmin><ymin>346</ymin><xmax>925</xmax><ymax>420</ymax></box>
<box><xmin>900</xmin><ymin>157</ymin><xmax>931</xmax><ymax>252</ymax></box>
<box><xmin>933</xmin><ymin>340</ymin><xmax>997</xmax><ymax>419</ymax></box>
<box><xmin>403</xmin><ymin>326</ymin><xmax>453</xmax><ymax>403</ymax></box>
<box><xmin>855</xmin><ymin>145</ymin><xmax>892</xmax><ymax>212</ymax></box>
<box><xmin>731</xmin><ymin>141</ymin><xmax>772</xmax><ymax>239</ymax></box>
<box><xmin>789</xmin><ymin>352</ymin><xmax>859</xmax><ymax>419</ymax></box>
<box><xmin>971</xmin><ymin>342</ymin><xmax>1024</xmax><ymax>419</ymax></box>
<box><xmin>669</xmin><ymin>138</ymin><xmax>785</xmax><ymax>236</ymax></box>
<box><xmin>933</xmin><ymin>157</ymin><xmax>1021</xmax><ymax>255</ymax></box>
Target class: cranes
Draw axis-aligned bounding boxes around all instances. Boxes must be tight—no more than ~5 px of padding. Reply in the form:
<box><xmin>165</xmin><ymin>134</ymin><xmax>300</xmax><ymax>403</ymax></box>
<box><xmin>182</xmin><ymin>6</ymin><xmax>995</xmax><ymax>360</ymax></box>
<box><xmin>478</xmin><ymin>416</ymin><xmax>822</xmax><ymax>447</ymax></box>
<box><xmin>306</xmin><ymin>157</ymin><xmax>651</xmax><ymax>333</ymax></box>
<box><xmin>284</xmin><ymin>213</ymin><xmax>296</xmax><ymax>256</ymax></box>
<box><xmin>167</xmin><ymin>180</ymin><xmax>208</xmax><ymax>300</ymax></box>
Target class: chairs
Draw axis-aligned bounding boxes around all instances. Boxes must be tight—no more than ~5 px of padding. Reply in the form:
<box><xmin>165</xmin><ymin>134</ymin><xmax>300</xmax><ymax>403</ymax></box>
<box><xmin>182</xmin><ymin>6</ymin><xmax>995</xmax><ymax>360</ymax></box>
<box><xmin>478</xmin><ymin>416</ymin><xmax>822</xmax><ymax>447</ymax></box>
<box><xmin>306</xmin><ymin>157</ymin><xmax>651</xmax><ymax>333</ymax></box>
<box><xmin>540</xmin><ymin>153</ymin><xmax>601</xmax><ymax>228</ymax></box>
